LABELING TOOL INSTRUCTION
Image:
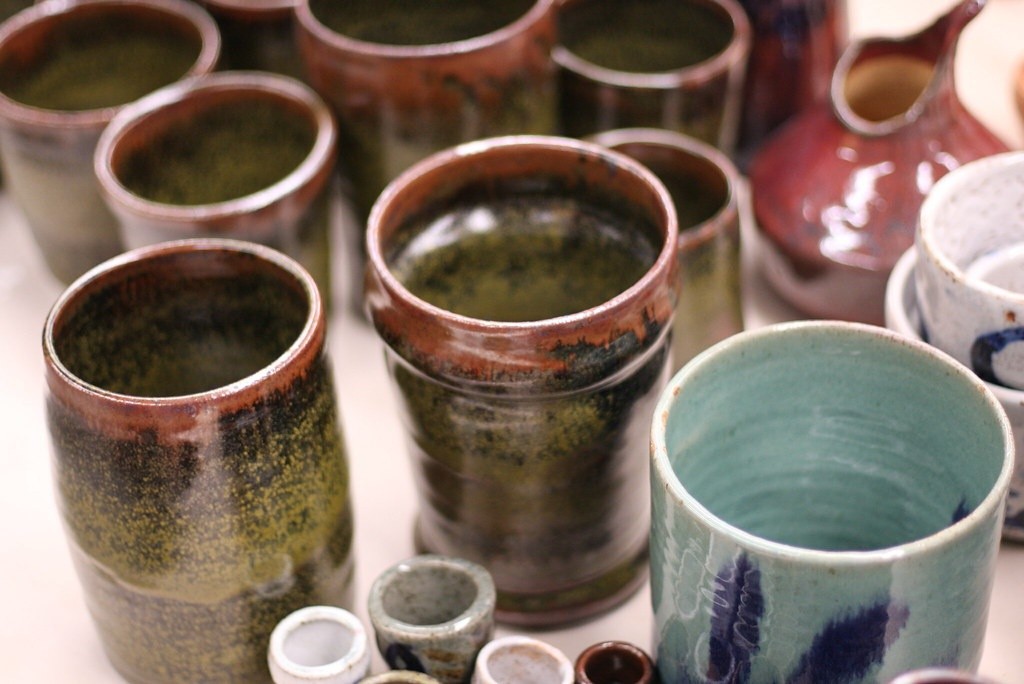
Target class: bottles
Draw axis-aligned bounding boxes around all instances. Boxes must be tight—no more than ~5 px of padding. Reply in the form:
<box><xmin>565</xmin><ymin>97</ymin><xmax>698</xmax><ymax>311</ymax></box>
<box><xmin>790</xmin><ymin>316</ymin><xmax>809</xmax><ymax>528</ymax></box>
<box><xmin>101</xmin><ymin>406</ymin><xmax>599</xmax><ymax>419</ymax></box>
<box><xmin>747</xmin><ymin>0</ymin><xmax>1015</xmax><ymax>322</ymax></box>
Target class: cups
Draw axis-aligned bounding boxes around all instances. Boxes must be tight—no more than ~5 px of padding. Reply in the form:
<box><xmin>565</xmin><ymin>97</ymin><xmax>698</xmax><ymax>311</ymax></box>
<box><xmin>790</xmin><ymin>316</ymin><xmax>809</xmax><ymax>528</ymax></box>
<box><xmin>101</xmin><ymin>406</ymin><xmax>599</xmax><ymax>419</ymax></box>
<box><xmin>41</xmin><ymin>239</ymin><xmax>357</xmax><ymax>684</ymax></box>
<box><xmin>647</xmin><ymin>318</ymin><xmax>1016</xmax><ymax>684</ymax></box>
<box><xmin>292</xmin><ymin>0</ymin><xmax>567</xmax><ymax>221</ymax></box>
<box><xmin>267</xmin><ymin>605</ymin><xmax>372</xmax><ymax>684</ymax></box>
<box><xmin>575</xmin><ymin>640</ymin><xmax>653</xmax><ymax>684</ymax></box>
<box><xmin>586</xmin><ymin>127</ymin><xmax>746</xmax><ymax>375</ymax></box>
<box><xmin>364</xmin><ymin>135</ymin><xmax>681</xmax><ymax>630</ymax></box>
<box><xmin>470</xmin><ymin>636</ymin><xmax>575</xmax><ymax>684</ymax></box>
<box><xmin>880</xmin><ymin>145</ymin><xmax>1024</xmax><ymax>547</ymax></box>
<box><xmin>92</xmin><ymin>67</ymin><xmax>338</xmax><ymax>332</ymax></box>
<box><xmin>0</xmin><ymin>0</ymin><xmax>221</xmax><ymax>285</ymax></box>
<box><xmin>367</xmin><ymin>554</ymin><xmax>497</xmax><ymax>684</ymax></box>
<box><xmin>553</xmin><ymin>0</ymin><xmax>752</xmax><ymax>167</ymax></box>
<box><xmin>734</xmin><ymin>0</ymin><xmax>854</xmax><ymax>175</ymax></box>
